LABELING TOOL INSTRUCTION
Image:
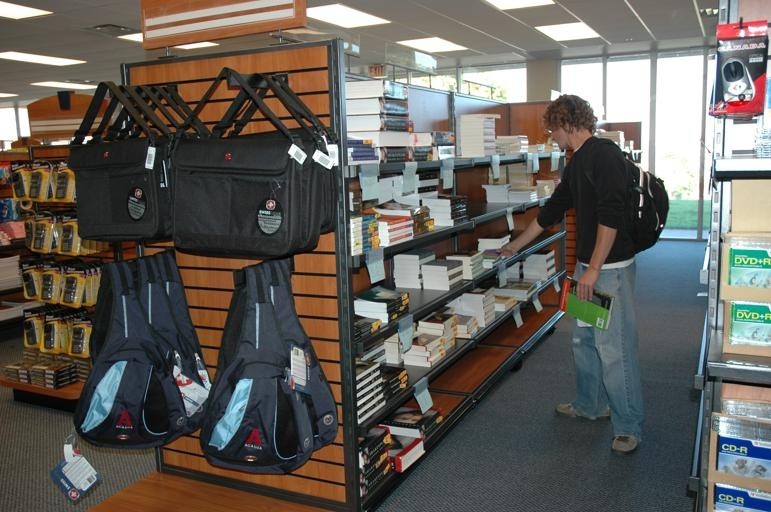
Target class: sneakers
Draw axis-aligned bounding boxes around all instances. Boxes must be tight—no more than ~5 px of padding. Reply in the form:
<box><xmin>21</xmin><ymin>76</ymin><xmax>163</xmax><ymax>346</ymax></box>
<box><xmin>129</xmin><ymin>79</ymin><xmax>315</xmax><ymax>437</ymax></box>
<box><xmin>556</xmin><ymin>403</ymin><xmax>610</xmax><ymax>420</ymax></box>
<box><xmin>612</xmin><ymin>435</ymin><xmax>639</xmax><ymax>453</ymax></box>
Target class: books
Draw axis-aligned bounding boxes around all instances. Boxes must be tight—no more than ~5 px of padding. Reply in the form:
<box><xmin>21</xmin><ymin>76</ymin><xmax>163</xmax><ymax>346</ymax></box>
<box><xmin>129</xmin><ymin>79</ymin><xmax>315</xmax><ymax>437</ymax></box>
<box><xmin>349</xmin><ymin>172</ymin><xmax>469</xmax><ymax>255</ymax></box>
<box><xmin>486</xmin><ymin>162</ymin><xmax>555</xmax><ymax>204</ymax></box>
<box><xmin>357</xmin><ymin>407</ymin><xmax>441</xmax><ymax>499</ymax></box>
<box><xmin>353</xmin><ymin>232</ymin><xmax>512</xmax><ymax>341</ymax></box>
<box><xmin>343</xmin><ymin>78</ymin><xmax>455</xmax><ymax>162</ymax></box>
<box><xmin>356</xmin><ymin>249</ymin><xmax>556</xmax><ymax>424</ymax></box>
<box><xmin>463</xmin><ymin>114</ymin><xmax>560</xmax><ymax>158</ymax></box>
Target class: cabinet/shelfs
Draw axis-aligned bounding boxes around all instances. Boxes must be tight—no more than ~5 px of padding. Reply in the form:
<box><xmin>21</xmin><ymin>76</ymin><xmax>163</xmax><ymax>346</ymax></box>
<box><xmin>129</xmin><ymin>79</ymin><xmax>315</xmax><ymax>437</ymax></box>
<box><xmin>684</xmin><ymin>0</ymin><xmax>770</xmax><ymax>512</ymax></box>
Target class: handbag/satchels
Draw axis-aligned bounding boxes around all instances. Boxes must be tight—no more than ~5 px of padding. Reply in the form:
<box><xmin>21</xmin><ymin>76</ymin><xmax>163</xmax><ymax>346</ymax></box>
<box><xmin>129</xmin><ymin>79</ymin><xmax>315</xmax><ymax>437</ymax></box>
<box><xmin>68</xmin><ymin>126</ymin><xmax>339</xmax><ymax>259</ymax></box>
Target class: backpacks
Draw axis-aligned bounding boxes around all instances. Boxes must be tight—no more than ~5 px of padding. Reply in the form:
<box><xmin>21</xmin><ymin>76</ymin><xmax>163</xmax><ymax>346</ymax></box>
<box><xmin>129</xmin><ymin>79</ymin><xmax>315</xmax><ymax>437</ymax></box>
<box><xmin>580</xmin><ymin>138</ymin><xmax>669</xmax><ymax>254</ymax></box>
